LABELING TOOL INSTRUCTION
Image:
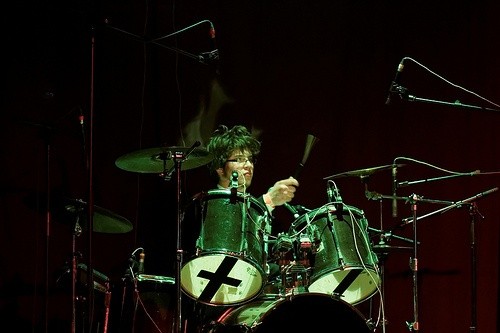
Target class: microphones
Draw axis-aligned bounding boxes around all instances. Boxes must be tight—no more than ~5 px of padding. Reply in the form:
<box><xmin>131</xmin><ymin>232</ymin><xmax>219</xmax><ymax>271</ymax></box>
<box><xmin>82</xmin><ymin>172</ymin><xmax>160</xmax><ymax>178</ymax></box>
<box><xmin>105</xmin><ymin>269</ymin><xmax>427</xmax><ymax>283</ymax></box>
<box><xmin>391</xmin><ymin>164</ymin><xmax>399</xmax><ymax>218</ymax></box>
<box><xmin>209</xmin><ymin>30</ymin><xmax>222</xmax><ymax>74</ymax></box>
<box><xmin>385</xmin><ymin>63</ymin><xmax>405</xmax><ymax>106</ymax></box>
<box><xmin>136</xmin><ymin>252</ymin><xmax>145</xmax><ymax>288</ymax></box>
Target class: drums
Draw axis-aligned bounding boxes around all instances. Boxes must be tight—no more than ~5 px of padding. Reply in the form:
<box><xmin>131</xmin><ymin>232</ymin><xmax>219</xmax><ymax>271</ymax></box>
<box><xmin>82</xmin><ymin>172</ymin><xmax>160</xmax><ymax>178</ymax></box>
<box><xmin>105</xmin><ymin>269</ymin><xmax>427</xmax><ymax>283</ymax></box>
<box><xmin>179</xmin><ymin>189</ymin><xmax>271</xmax><ymax>307</ymax></box>
<box><xmin>118</xmin><ymin>272</ymin><xmax>196</xmax><ymax>333</ymax></box>
<box><xmin>286</xmin><ymin>205</ymin><xmax>382</xmax><ymax>306</ymax></box>
<box><xmin>204</xmin><ymin>291</ymin><xmax>376</xmax><ymax>333</ymax></box>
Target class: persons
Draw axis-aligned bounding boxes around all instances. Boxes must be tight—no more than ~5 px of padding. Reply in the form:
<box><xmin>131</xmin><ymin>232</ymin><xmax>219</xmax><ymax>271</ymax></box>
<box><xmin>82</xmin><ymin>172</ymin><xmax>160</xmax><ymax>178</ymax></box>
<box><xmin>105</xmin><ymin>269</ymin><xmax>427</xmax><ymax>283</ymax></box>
<box><xmin>192</xmin><ymin>126</ymin><xmax>298</xmax><ymax>217</ymax></box>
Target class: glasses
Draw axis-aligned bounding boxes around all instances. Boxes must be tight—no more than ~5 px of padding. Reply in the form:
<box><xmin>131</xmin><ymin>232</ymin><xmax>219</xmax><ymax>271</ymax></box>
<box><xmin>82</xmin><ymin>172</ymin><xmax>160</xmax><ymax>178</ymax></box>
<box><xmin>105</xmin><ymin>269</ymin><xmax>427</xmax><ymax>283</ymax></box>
<box><xmin>227</xmin><ymin>155</ymin><xmax>257</xmax><ymax>165</ymax></box>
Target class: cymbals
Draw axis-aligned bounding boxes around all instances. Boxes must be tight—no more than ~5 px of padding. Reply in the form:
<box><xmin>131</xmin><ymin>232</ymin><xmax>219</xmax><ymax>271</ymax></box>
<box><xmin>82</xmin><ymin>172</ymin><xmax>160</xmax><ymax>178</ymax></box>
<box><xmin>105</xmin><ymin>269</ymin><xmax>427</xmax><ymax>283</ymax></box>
<box><xmin>115</xmin><ymin>146</ymin><xmax>214</xmax><ymax>174</ymax></box>
<box><xmin>322</xmin><ymin>163</ymin><xmax>405</xmax><ymax>180</ymax></box>
<box><xmin>55</xmin><ymin>200</ymin><xmax>133</xmax><ymax>234</ymax></box>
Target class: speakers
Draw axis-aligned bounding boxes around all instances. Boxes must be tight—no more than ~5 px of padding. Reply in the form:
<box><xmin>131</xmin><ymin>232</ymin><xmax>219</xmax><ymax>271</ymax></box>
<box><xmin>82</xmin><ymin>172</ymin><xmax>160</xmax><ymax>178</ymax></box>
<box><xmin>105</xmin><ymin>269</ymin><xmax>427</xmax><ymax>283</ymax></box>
<box><xmin>112</xmin><ymin>289</ymin><xmax>189</xmax><ymax>333</ymax></box>
